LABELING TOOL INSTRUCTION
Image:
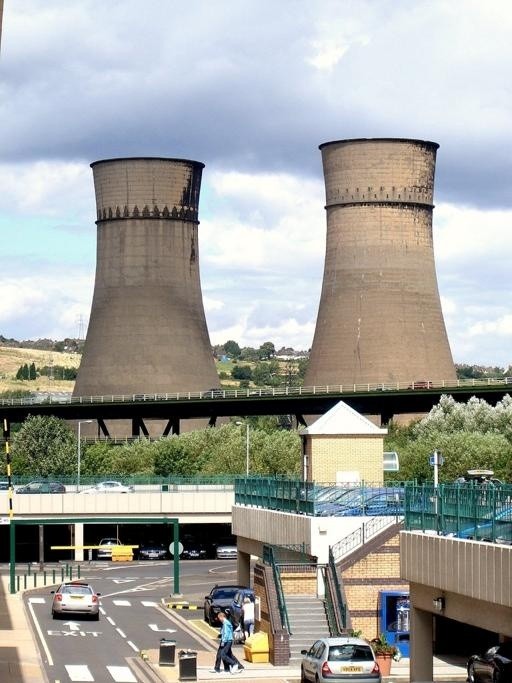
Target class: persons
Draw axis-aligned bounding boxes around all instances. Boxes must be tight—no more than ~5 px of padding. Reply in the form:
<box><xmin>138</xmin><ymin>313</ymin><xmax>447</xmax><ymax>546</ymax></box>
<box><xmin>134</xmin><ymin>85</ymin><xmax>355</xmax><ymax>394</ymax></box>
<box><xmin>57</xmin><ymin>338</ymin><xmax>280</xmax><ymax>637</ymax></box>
<box><xmin>218</xmin><ymin>613</ymin><xmax>239</xmax><ymax>675</ymax></box>
<box><xmin>241</xmin><ymin>598</ymin><xmax>255</xmax><ymax>639</ymax></box>
<box><xmin>209</xmin><ymin>609</ymin><xmax>244</xmax><ymax>674</ymax></box>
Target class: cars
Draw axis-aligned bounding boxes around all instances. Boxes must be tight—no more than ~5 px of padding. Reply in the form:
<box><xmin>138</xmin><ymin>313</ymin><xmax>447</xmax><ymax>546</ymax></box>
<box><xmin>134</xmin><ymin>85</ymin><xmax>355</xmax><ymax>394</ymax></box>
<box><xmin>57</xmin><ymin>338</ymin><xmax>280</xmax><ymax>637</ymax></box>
<box><xmin>212</xmin><ymin>542</ymin><xmax>238</xmax><ymax>559</ymax></box>
<box><xmin>249</xmin><ymin>390</ymin><xmax>271</xmax><ymax>396</ymax></box>
<box><xmin>0</xmin><ymin>481</ymin><xmax>15</xmax><ymax>492</ymax></box>
<box><xmin>452</xmin><ymin>504</ymin><xmax>512</xmax><ymax>545</ymax></box>
<box><xmin>16</xmin><ymin>480</ymin><xmax>66</xmax><ymax>493</ymax></box>
<box><xmin>250</xmin><ymin>486</ymin><xmax>433</xmax><ymax>516</ymax></box>
<box><xmin>204</xmin><ymin>583</ymin><xmax>254</xmax><ymax>626</ymax></box>
<box><xmin>181</xmin><ymin>534</ymin><xmax>209</xmax><ymax>559</ymax></box>
<box><xmin>129</xmin><ymin>393</ymin><xmax>149</xmax><ymax>402</ymax></box>
<box><xmin>138</xmin><ymin>537</ymin><xmax>171</xmax><ymax>560</ymax></box>
<box><xmin>79</xmin><ymin>480</ymin><xmax>135</xmax><ymax>493</ymax></box>
<box><xmin>299</xmin><ymin>635</ymin><xmax>381</xmax><ymax>682</ymax></box>
<box><xmin>201</xmin><ymin>388</ymin><xmax>223</xmax><ymax>399</ymax></box>
<box><xmin>49</xmin><ymin>582</ymin><xmax>103</xmax><ymax>620</ymax></box>
<box><xmin>368</xmin><ymin>387</ymin><xmax>398</xmax><ymax>391</ymax></box>
<box><xmin>408</xmin><ymin>380</ymin><xmax>432</xmax><ymax>390</ymax></box>
<box><xmin>466</xmin><ymin>643</ymin><xmax>512</xmax><ymax>682</ymax></box>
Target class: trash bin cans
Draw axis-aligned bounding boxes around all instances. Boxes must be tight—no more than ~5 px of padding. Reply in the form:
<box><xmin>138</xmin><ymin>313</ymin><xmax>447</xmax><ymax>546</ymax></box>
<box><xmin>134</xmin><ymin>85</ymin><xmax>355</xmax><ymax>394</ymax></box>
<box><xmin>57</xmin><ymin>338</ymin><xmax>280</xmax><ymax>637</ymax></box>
<box><xmin>178</xmin><ymin>653</ymin><xmax>197</xmax><ymax>680</ymax></box>
<box><xmin>159</xmin><ymin>640</ymin><xmax>175</xmax><ymax>665</ymax></box>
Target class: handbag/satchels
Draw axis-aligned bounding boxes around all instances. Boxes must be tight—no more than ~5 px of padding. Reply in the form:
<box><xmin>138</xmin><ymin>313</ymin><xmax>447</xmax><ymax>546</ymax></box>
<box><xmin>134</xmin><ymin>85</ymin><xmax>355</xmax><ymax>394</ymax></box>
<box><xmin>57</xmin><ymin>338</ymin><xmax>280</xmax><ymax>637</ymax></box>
<box><xmin>231</xmin><ymin>623</ymin><xmax>246</xmax><ymax>642</ymax></box>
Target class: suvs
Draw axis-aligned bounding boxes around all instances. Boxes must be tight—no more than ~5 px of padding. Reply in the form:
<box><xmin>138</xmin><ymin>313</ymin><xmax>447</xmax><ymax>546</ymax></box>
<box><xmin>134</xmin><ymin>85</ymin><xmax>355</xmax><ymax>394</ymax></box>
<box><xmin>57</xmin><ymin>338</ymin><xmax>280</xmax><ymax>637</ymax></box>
<box><xmin>96</xmin><ymin>537</ymin><xmax>126</xmax><ymax>559</ymax></box>
<box><xmin>454</xmin><ymin>469</ymin><xmax>502</xmax><ymax>483</ymax></box>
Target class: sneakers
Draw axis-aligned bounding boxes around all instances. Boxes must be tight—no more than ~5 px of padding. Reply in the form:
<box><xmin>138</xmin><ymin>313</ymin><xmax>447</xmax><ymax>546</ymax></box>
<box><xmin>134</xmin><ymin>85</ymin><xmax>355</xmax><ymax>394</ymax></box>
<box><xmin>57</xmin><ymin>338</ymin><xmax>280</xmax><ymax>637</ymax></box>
<box><xmin>208</xmin><ymin>663</ymin><xmax>244</xmax><ymax>675</ymax></box>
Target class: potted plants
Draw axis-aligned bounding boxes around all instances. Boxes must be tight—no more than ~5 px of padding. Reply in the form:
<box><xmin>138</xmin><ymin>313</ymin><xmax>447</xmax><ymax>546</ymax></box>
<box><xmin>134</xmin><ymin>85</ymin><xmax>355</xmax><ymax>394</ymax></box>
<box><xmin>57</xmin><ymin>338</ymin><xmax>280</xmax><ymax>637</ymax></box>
<box><xmin>371</xmin><ymin>634</ymin><xmax>401</xmax><ymax>676</ymax></box>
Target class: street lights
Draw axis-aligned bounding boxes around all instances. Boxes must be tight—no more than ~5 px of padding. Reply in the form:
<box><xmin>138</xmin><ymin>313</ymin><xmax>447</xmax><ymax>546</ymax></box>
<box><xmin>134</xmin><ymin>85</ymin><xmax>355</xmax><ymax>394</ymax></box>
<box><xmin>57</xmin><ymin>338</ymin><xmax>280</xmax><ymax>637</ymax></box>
<box><xmin>236</xmin><ymin>421</ymin><xmax>251</xmax><ymax>478</ymax></box>
<box><xmin>77</xmin><ymin>419</ymin><xmax>93</xmax><ymax>491</ymax></box>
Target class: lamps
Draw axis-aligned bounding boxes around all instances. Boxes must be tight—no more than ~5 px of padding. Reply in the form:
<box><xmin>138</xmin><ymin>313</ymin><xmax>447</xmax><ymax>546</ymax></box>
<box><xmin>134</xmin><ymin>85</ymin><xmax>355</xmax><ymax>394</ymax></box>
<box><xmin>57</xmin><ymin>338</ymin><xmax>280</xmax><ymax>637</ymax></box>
<box><xmin>431</xmin><ymin>596</ymin><xmax>444</xmax><ymax>613</ymax></box>
